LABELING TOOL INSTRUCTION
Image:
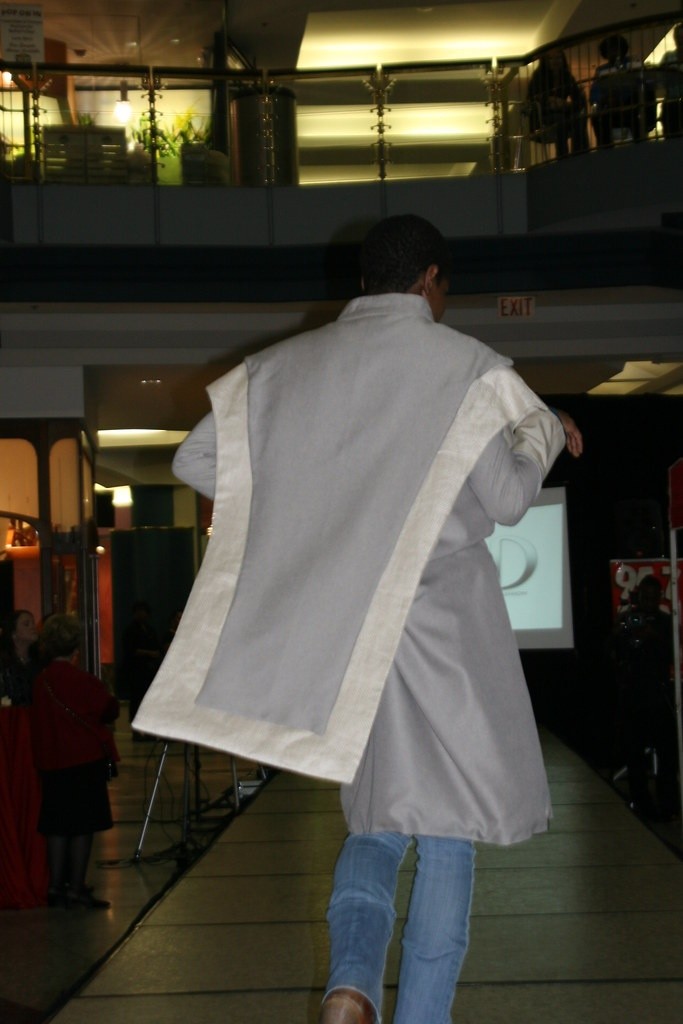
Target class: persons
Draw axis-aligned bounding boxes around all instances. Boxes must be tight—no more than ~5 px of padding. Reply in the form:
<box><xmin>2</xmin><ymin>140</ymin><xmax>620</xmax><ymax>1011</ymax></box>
<box><xmin>122</xmin><ymin>602</ymin><xmax>159</xmax><ymax>741</ymax></box>
<box><xmin>133</xmin><ymin>214</ymin><xmax>583</xmax><ymax>1024</ymax></box>
<box><xmin>166</xmin><ymin>609</ymin><xmax>182</xmax><ymax>639</ymax></box>
<box><xmin>0</xmin><ymin>610</ymin><xmax>120</xmax><ymax>909</ymax></box>
<box><xmin>525</xmin><ymin>46</ymin><xmax>587</xmax><ymax>157</ymax></box>
<box><xmin>590</xmin><ymin>35</ymin><xmax>656</xmax><ymax>144</ymax></box>
<box><xmin>609</xmin><ymin>576</ymin><xmax>683</xmax><ymax>823</ymax></box>
<box><xmin>661</xmin><ymin>23</ymin><xmax>683</xmax><ymax>134</ymax></box>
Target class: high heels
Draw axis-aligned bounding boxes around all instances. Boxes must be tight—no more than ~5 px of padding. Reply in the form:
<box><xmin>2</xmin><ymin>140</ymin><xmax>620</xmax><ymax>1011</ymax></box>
<box><xmin>47</xmin><ymin>884</ymin><xmax>96</xmax><ymax>908</ymax></box>
<box><xmin>66</xmin><ymin>889</ymin><xmax>110</xmax><ymax>910</ymax></box>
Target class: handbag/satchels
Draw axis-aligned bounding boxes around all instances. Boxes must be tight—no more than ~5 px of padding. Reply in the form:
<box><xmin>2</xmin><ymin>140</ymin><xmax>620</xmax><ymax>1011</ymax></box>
<box><xmin>104</xmin><ymin>746</ymin><xmax>119</xmax><ymax>778</ymax></box>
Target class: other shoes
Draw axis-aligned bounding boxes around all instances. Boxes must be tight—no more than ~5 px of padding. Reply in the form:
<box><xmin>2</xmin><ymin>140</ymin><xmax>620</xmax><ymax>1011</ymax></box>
<box><xmin>321</xmin><ymin>989</ymin><xmax>377</xmax><ymax>1024</ymax></box>
